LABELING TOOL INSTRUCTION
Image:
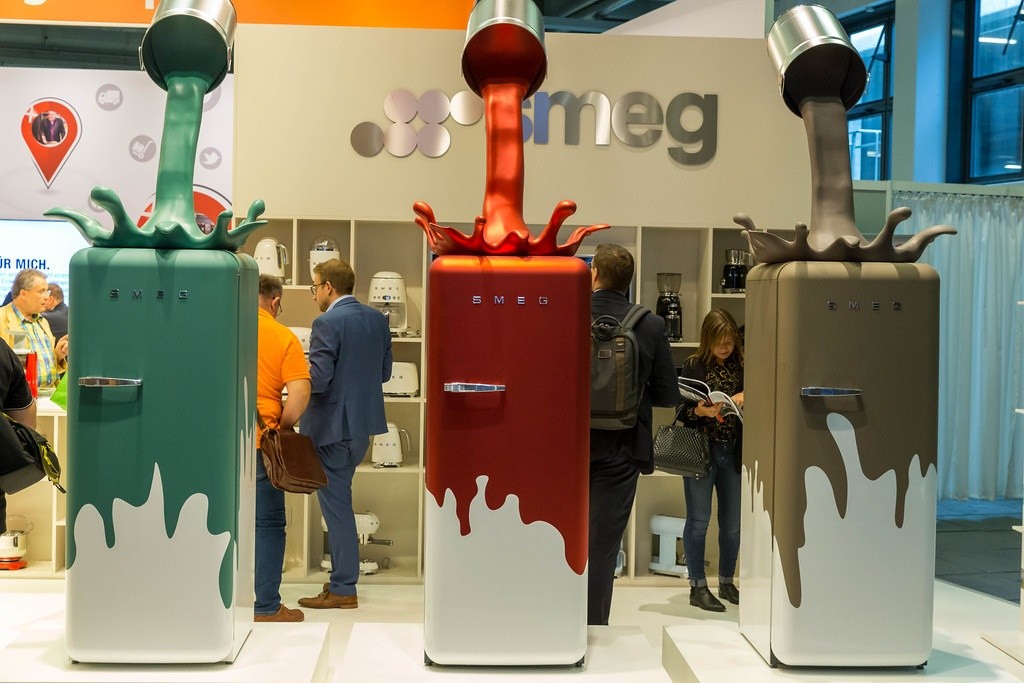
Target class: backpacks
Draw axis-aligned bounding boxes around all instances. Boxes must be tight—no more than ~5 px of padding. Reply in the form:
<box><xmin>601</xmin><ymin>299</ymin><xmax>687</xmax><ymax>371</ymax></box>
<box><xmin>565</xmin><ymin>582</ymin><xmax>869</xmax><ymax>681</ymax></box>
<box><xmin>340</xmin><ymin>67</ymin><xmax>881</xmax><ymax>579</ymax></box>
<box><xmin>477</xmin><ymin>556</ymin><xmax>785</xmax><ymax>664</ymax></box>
<box><xmin>587</xmin><ymin>303</ymin><xmax>651</xmax><ymax>430</ymax></box>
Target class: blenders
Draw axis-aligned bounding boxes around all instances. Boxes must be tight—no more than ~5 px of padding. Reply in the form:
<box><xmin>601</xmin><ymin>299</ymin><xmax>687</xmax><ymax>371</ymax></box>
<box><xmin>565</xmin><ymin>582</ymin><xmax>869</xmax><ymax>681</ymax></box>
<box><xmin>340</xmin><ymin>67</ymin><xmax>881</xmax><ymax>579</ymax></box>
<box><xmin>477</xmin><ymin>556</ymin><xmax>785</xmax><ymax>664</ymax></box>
<box><xmin>654</xmin><ymin>271</ymin><xmax>686</xmax><ymax>343</ymax></box>
<box><xmin>319</xmin><ymin>513</ymin><xmax>393</xmax><ymax>576</ymax></box>
<box><xmin>647</xmin><ymin>514</ymin><xmax>711</xmax><ymax>581</ymax></box>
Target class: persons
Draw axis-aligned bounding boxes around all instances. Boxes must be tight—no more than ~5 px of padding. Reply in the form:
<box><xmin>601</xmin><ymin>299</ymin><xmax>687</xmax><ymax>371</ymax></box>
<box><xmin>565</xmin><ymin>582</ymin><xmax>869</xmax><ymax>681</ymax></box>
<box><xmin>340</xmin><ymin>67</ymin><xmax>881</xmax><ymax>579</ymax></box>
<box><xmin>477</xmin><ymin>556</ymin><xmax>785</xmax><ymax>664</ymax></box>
<box><xmin>0</xmin><ymin>269</ymin><xmax>69</xmax><ymax>535</ymax></box>
<box><xmin>255</xmin><ymin>274</ymin><xmax>311</xmax><ymax>621</ymax></box>
<box><xmin>675</xmin><ymin>307</ymin><xmax>743</xmax><ymax>611</ymax></box>
<box><xmin>298</xmin><ymin>259</ymin><xmax>392</xmax><ymax>609</ymax></box>
<box><xmin>587</xmin><ymin>244</ymin><xmax>679</xmax><ymax>626</ymax></box>
<box><xmin>40</xmin><ymin>111</ymin><xmax>65</xmax><ymax>144</ymax></box>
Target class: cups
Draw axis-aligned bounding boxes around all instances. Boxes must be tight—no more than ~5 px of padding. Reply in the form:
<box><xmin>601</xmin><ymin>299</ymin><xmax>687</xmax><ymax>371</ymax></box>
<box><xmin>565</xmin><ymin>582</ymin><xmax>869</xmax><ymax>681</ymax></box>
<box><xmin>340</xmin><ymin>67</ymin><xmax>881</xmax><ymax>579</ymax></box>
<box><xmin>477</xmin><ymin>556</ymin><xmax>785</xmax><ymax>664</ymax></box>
<box><xmin>308</xmin><ymin>237</ymin><xmax>341</xmax><ymax>282</ymax></box>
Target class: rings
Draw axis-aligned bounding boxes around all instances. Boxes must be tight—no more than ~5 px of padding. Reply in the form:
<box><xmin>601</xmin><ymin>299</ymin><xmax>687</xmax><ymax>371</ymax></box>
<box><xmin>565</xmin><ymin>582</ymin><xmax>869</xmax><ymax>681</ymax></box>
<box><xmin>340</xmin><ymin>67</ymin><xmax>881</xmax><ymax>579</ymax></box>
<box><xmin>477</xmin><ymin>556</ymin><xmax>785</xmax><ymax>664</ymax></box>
<box><xmin>714</xmin><ymin>411</ymin><xmax>717</xmax><ymax>415</ymax></box>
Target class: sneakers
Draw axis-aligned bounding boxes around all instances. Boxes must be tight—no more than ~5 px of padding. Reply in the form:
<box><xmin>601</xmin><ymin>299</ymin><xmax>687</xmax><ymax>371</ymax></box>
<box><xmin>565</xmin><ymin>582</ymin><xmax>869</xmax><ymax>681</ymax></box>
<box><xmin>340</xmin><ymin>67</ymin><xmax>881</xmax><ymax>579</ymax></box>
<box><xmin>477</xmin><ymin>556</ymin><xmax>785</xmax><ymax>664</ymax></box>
<box><xmin>298</xmin><ymin>582</ymin><xmax>359</xmax><ymax>609</ymax></box>
<box><xmin>254</xmin><ymin>603</ymin><xmax>304</xmax><ymax>622</ymax></box>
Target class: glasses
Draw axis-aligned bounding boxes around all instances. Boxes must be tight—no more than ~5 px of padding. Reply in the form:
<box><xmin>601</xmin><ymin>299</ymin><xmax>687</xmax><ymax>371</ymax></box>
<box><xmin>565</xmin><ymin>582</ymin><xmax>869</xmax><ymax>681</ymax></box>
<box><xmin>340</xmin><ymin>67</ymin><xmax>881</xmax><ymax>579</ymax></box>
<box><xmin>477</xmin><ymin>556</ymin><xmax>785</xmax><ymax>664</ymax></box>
<box><xmin>26</xmin><ymin>289</ymin><xmax>51</xmax><ymax>297</ymax></box>
<box><xmin>587</xmin><ymin>262</ymin><xmax>597</xmax><ymax>269</ymax></box>
<box><xmin>310</xmin><ymin>282</ymin><xmax>335</xmax><ymax>294</ymax></box>
<box><xmin>272</xmin><ymin>296</ymin><xmax>283</xmax><ymax>316</ymax></box>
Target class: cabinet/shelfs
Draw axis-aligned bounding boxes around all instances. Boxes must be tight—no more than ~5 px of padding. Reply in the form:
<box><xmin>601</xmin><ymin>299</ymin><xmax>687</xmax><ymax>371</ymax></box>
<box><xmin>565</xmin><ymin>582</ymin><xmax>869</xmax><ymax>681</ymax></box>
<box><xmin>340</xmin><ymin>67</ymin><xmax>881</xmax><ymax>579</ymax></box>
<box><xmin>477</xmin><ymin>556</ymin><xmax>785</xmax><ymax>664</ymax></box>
<box><xmin>234</xmin><ymin>217</ymin><xmax>916</xmax><ymax>590</ymax></box>
<box><xmin>0</xmin><ymin>395</ymin><xmax>67</xmax><ymax>581</ymax></box>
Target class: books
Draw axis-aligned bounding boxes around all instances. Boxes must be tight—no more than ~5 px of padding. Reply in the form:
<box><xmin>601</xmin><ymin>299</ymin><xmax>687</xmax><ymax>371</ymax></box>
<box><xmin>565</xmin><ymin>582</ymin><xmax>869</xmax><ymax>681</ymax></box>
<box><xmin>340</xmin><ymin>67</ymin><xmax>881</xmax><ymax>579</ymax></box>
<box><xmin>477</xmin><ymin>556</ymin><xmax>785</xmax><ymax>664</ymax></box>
<box><xmin>678</xmin><ymin>377</ymin><xmax>743</xmax><ymax>424</ymax></box>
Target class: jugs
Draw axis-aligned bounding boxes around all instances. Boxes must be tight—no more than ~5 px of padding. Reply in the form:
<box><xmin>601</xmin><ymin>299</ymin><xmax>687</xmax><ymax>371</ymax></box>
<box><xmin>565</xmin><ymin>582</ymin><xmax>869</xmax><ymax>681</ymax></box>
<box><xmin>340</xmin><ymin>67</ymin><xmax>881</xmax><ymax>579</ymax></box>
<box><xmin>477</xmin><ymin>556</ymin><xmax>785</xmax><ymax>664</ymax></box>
<box><xmin>6</xmin><ymin>329</ymin><xmax>39</xmax><ymax>399</ymax></box>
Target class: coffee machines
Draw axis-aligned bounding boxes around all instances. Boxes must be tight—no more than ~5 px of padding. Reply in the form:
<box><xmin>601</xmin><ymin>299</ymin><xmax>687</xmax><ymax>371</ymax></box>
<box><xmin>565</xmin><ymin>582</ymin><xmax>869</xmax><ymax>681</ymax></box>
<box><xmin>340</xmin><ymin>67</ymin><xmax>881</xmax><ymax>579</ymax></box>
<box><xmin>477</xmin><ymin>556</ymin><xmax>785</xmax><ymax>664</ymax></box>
<box><xmin>366</xmin><ymin>270</ymin><xmax>410</xmax><ymax>334</ymax></box>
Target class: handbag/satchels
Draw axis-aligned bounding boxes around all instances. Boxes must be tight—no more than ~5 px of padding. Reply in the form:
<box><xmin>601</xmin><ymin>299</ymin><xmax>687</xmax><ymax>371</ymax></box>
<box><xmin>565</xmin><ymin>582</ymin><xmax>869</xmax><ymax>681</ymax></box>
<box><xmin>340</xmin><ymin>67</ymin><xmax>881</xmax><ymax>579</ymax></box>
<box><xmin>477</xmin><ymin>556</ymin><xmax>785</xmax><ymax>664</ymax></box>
<box><xmin>651</xmin><ymin>403</ymin><xmax>712</xmax><ymax>480</ymax></box>
<box><xmin>1</xmin><ymin>412</ymin><xmax>66</xmax><ymax>495</ymax></box>
<box><xmin>259</xmin><ymin>427</ymin><xmax>328</xmax><ymax>496</ymax></box>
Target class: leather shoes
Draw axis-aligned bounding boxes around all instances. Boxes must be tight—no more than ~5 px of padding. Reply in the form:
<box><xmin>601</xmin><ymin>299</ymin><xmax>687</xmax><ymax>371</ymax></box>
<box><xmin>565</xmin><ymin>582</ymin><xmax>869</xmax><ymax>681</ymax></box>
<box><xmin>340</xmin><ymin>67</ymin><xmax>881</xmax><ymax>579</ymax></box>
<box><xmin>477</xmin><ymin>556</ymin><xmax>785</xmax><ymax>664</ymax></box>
<box><xmin>689</xmin><ymin>586</ymin><xmax>726</xmax><ymax>612</ymax></box>
<box><xmin>718</xmin><ymin>583</ymin><xmax>739</xmax><ymax>605</ymax></box>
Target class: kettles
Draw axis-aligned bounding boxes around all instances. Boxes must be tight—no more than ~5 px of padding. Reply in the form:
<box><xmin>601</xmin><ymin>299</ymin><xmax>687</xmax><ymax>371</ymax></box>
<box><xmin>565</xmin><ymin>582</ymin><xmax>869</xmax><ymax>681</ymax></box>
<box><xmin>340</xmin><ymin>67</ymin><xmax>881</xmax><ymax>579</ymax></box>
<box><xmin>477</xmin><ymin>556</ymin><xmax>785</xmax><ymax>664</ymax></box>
<box><xmin>720</xmin><ymin>250</ymin><xmax>755</xmax><ymax>295</ymax></box>
<box><xmin>253</xmin><ymin>236</ymin><xmax>290</xmax><ymax>285</ymax></box>
<box><xmin>0</xmin><ymin>515</ymin><xmax>34</xmax><ymax>562</ymax></box>
<box><xmin>372</xmin><ymin>422</ymin><xmax>413</xmax><ymax>469</ymax></box>
